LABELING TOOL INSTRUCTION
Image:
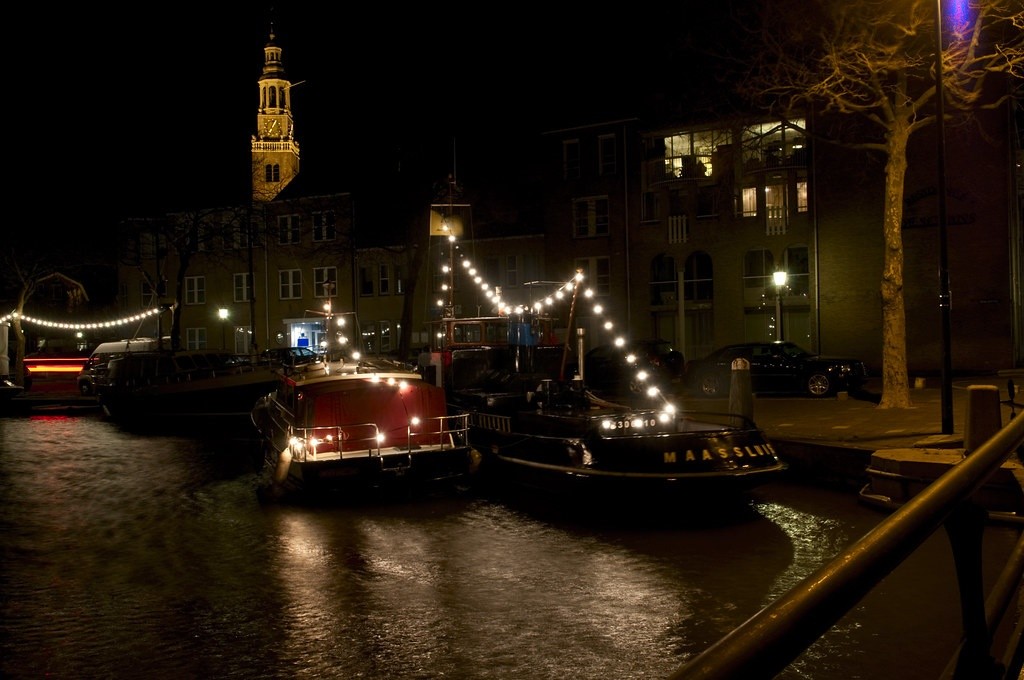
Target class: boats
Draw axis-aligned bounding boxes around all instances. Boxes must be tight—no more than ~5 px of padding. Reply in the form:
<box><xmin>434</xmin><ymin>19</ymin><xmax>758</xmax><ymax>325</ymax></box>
<box><xmin>249</xmin><ymin>278</ymin><xmax>474</xmax><ymax>502</ymax></box>
<box><xmin>74</xmin><ymin>284</ymin><xmax>326</xmax><ymax>419</ymax></box>
<box><xmin>413</xmin><ymin>177</ymin><xmax>796</xmax><ymax>513</ymax></box>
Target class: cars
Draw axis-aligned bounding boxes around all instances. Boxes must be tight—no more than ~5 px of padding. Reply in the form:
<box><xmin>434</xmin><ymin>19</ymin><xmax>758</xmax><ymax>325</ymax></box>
<box><xmin>679</xmin><ymin>335</ymin><xmax>869</xmax><ymax>399</ymax></box>
<box><xmin>566</xmin><ymin>337</ymin><xmax>676</xmax><ymax>395</ymax></box>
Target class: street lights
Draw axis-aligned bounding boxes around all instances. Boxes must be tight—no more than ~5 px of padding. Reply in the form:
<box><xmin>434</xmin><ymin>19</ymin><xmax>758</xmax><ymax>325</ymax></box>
<box><xmin>768</xmin><ymin>266</ymin><xmax>790</xmax><ymax>340</ymax></box>
<box><xmin>216</xmin><ymin>307</ymin><xmax>231</xmax><ymax>350</ymax></box>
